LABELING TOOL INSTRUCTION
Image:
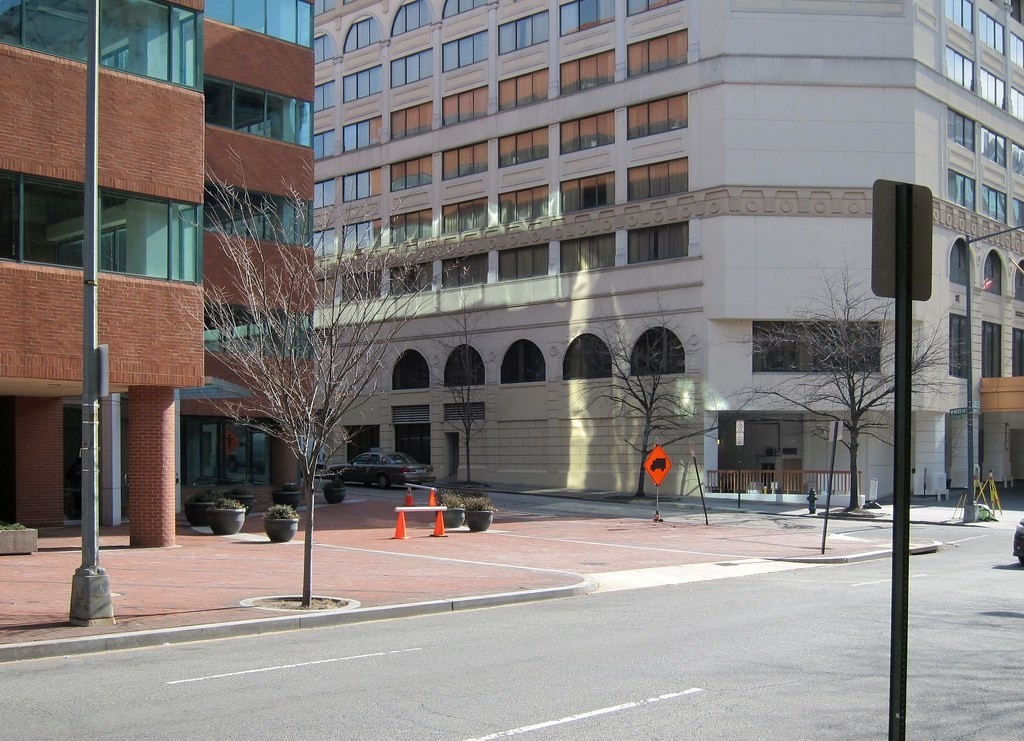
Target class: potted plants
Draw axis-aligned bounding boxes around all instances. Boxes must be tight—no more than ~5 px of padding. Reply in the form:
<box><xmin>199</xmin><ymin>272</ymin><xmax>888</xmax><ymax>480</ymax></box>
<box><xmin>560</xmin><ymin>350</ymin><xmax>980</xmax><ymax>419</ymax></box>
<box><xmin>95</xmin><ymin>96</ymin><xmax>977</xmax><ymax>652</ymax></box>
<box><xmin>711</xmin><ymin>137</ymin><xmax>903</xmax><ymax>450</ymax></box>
<box><xmin>272</xmin><ymin>482</ymin><xmax>302</xmax><ymax>510</ymax></box>
<box><xmin>262</xmin><ymin>503</ymin><xmax>303</xmax><ymax>543</ymax></box>
<box><xmin>206</xmin><ymin>497</ymin><xmax>250</xmax><ymax>535</ymax></box>
<box><xmin>321</xmin><ymin>474</ymin><xmax>348</xmax><ymax>504</ymax></box>
<box><xmin>0</xmin><ymin>521</ymin><xmax>39</xmax><ymax>554</ymax></box>
<box><xmin>225</xmin><ymin>487</ymin><xmax>254</xmax><ymax>516</ymax></box>
<box><xmin>183</xmin><ymin>491</ymin><xmax>224</xmax><ymax>526</ymax></box>
<box><xmin>434</xmin><ymin>488</ymin><xmax>465</xmax><ymax>528</ymax></box>
<box><xmin>462</xmin><ymin>495</ymin><xmax>498</xmax><ymax>531</ymax></box>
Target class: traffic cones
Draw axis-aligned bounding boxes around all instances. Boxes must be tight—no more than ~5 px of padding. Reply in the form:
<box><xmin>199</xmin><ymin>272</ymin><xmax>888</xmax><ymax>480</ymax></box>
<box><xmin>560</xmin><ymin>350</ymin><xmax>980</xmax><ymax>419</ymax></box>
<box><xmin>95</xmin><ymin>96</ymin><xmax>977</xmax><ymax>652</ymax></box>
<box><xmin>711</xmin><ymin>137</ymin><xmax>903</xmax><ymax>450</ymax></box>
<box><xmin>428</xmin><ymin>509</ymin><xmax>449</xmax><ymax>538</ymax></box>
<box><xmin>403</xmin><ymin>485</ymin><xmax>416</xmax><ymax>508</ymax></box>
<box><xmin>392</xmin><ymin>509</ymin><xmax>411</xmax><ymax>539</ymax></box>
<box><xmin>428</xmin><ymin>488</ymin><xmax>438</xmax><ymax>507</ymax></box>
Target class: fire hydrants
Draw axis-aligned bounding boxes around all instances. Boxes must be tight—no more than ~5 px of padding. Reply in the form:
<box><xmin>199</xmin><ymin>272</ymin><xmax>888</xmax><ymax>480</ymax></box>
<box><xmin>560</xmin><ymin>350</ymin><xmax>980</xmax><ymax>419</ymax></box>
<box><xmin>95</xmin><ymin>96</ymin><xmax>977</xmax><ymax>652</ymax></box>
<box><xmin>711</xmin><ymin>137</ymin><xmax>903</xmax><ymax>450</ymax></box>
<box><xmin>806</xmin><ymin>487</ymin><xmax>819</xmax><ymax>514</ymax></box>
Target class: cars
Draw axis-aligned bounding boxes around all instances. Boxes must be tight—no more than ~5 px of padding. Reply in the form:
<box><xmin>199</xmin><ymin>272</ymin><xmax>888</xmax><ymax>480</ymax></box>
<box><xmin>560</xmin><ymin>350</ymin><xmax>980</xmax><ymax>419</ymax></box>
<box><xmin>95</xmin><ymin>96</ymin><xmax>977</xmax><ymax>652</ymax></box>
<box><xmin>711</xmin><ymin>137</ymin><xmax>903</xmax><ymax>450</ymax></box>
<box><xmin>328</xmin><ymin>449</ymin><xmax>437</xmax><ymax>490</ymax></box>
